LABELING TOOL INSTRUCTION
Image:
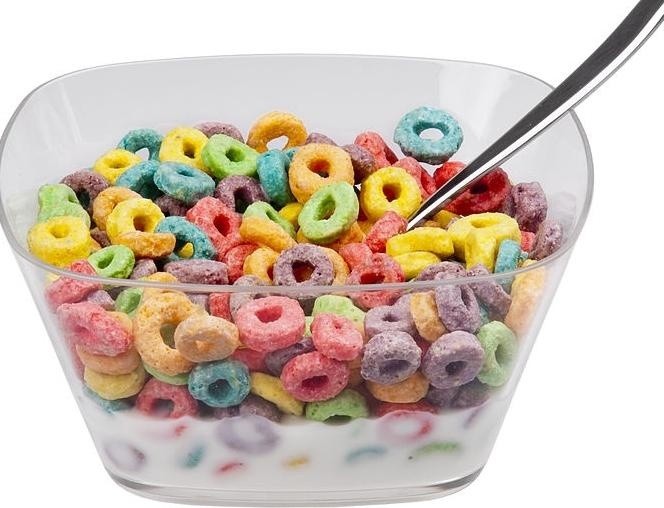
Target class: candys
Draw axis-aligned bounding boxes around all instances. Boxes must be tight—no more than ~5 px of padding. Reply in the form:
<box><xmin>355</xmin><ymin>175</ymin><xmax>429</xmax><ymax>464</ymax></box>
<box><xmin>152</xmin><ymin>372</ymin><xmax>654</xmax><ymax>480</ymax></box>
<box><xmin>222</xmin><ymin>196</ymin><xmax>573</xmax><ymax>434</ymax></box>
<box><xmin>29</xmin><ymin>106</ymin><xmax>563</xmax><ymax>478</ymax></box>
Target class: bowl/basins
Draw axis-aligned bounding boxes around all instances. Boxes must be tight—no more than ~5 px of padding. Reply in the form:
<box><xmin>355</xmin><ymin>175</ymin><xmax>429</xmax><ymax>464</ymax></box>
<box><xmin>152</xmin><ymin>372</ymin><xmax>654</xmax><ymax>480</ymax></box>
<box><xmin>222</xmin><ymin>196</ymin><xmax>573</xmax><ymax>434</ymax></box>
<box><xmin>0</xmin><ymin>51</ymin><xmax>596</xmax><ymax>508</ymax></box>
